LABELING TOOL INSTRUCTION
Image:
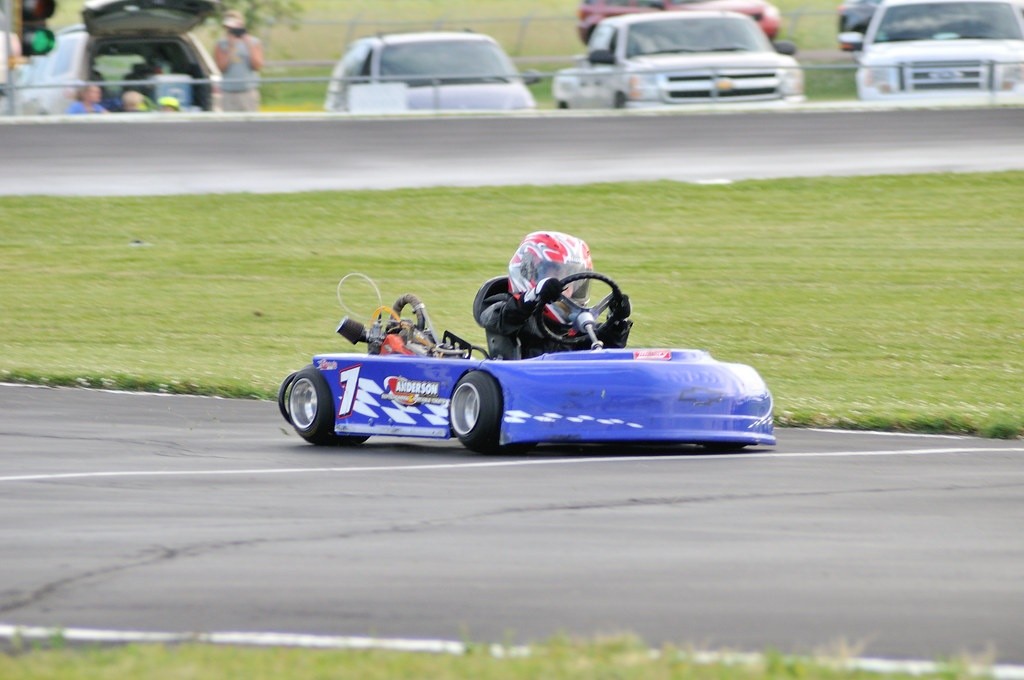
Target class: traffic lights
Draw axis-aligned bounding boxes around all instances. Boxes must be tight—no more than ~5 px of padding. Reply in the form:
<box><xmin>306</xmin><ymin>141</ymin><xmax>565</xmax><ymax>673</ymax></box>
<box><xmin>11</xmin><ymin>0</ymin><xmax>58</xmax><ymax>58</ymax></box>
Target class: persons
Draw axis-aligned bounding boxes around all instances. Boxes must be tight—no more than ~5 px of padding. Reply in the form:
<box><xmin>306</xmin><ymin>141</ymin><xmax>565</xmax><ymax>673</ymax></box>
<box><xmin>65</xmin><ymin>9</ymin><xmax>265</xmax><ymax>116</ymax></box>
<box><xmin>479</xmin><ymin>231</ymin><xmax>631</xmax><ymax>361</ymax></box>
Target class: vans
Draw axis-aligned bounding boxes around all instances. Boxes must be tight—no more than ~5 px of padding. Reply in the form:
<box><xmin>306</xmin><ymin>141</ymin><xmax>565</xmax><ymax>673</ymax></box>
<box><xmin>0</xmin><ymin>1</ymin><xmax>226</xmax><ymax>115</ymax></box>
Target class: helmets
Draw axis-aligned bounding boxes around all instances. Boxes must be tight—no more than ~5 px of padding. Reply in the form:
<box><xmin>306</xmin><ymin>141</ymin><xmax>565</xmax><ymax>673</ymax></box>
<box><xmin>508</xmin><ymin>231</ymin><xmax>593</xmax><ymax>325</ymax></box>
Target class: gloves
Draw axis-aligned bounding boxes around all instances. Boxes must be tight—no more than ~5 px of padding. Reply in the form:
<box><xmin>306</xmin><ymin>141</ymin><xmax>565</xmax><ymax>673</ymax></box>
<box><xmin>520</xmin><ymin>277</ymin><xmax>562</xmax><ymax>312</ymax></box>
<box><xmin>607</xmin><ymin>295</ymin><xmax>631</xmax><ymax>333</ymax></box>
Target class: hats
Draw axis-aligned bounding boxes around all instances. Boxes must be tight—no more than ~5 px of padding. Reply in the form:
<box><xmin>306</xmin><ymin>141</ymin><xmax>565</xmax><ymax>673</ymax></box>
<box><xmin>158</xmin><ymin>96</ymin><xmax>180</xmax><ymax>111</ymax></box>
<box><xmin>222</xmin><ymin>11</ymin><xmax>245</xmax><ymax>30</ymax></box>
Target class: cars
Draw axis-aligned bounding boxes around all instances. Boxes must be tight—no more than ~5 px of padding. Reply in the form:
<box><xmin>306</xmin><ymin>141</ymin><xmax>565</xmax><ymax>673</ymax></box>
<box><xmin>551</xmin><ymin>7</ymin><xmax>809</xmax><ymax>109</ymax></box>
<box><xmin>834</xmin><ymin>0</ymin><xmax>1024</xmax><ymax>102</ymax></box>
<box><xmin>575</xmin><ymin>0</ymin><xmax>784</xmax><ymax>45</ymax></box>
<box><xmin>322</xmin><ymin>28</ymin><xmax>546</xmax><ymax>114</ymax></box>
